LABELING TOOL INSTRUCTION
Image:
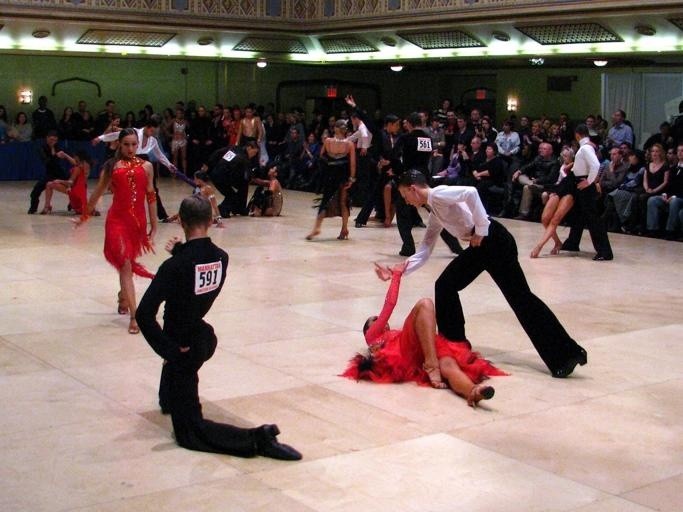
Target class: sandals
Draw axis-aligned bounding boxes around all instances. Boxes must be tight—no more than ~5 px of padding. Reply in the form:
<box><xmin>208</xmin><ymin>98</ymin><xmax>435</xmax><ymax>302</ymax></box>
<box><xmin>128</xmin><ymin>317</ymin><xmax>139</xmax><ymax>334</ymax></box>
<box><xmin>118</xmin><ymin>291</ymin><xmax>129</xmax><ymax>314</ymax></box>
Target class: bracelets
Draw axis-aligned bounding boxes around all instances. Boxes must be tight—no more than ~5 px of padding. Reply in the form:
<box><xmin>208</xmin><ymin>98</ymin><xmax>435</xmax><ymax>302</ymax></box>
<box><xmin>80</xmin><ymin>214</ymin><xmax>88</xmax><ymax>222</ymax></box>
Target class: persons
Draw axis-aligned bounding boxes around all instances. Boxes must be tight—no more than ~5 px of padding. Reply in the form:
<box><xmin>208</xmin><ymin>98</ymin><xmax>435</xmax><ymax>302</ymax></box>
<box><xmin>133</xmin><ymin>195</ymin><xmax>304</xmax><ymax>461</ymax></box>
<box><xmin>335</xmin><ymin>259</ymin><xmax>509</xmax><ymax>410</ymax></box>
<box><xmin>373</xmin><ymin>168</ymin><xmax>587</xmax><ymax>378</ymax></box>
<box><xmin>65</xmin><ymin>128</ymin><xmax>157</xmax><ymax>335</ymax></box>
<box><xmin>2</xmin><ymin>96</ymin><xmax>96</xmax><ymax>213</ymax></box>
<box><xmin>528</xmin><ymin>142</ymin><xmax>596</xmax><ymax>259</ymax></box>
<box><xmin>89</xmin><ymin>100</ymin><xmax>299</xmax><ymax>228</ymax></box>
<box><xmin>556</xmin><ymin>124</ymin><xmax>614</xmax><ymax>261</ymax></box>
<box><xmin>304</xmin><ymin>119</ymin><xmax>355</xmax><ymax>241</ymax></box>
<box><xmin>302</xmin><ymin>93</ymin><xmax>683</xmax><ymax>235</ymax></box>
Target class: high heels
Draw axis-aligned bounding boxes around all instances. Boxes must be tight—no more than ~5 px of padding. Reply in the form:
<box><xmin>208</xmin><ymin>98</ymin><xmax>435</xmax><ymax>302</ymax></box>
<box><xmin>550</xmin><ymin>241</ymin><xmax>562</xmax><ymax>255</ymax></box>
<box><xmin>40</xmin><ymin>206</ymin><xmax>52</xmax><ymax>214</ymax></box>
<box><xmin>337</xmin><ymin>230</ymin><xmax>348</xmax><ymax>240</ymax></box>
<box><xmin>467</xmin><ymin>385</ymin><xmax>495</xmax><ymax>409</ymax></box>
<box><xmin>422</xmin><ymin>364</ymin><xmax>448</xmax><ymax>389</ymax></box>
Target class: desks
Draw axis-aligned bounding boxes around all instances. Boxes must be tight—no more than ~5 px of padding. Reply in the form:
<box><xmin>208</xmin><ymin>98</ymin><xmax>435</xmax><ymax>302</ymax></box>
<box><xmin>0</xmin><ymin>139</ymin><xmax>173</xmax><ymax>181</ymax></box>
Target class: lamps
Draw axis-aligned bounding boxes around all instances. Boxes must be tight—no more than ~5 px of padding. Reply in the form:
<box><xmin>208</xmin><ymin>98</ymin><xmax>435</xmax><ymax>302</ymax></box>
<box><xmin>18</xmin><ymin>90</ymin><xmax>31</xmax><ymax>104</ymax></box>
<box><xmin>390</xmin><ymin>65</ymin><xmax>403</xmax><ymax>72</ymax></box>
<box><xmin>593</xmin><ymin>60</ymin><xmax>608</xmax><ymax>67</ymax></box>
<box><xmin>256</xmin><ymin>61</ymin><xmax>267</xmax><ymax>68</ymax></box>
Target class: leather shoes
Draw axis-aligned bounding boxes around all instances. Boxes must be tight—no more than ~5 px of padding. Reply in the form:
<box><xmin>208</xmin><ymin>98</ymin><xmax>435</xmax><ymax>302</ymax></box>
<box><xmin>552</xmin><ymin>346</ymin><xmax>587</xmax><ymax>377</ymax></box>
<box><xmin>593</xmin><ymin>253</ymin><xmax>604</xmax><ymax>261</ymax></box>
<box><xmin>28</xmin><ymin>208</ymin><xmax>37</xmax><ymax>214</ymax></box>
<box><xmin>416</xmin><ymin>222</ymin><xmax>427</xmax><ymax>228</ymax></box>
<box><xmin>255</xmin><ymin>424</ymin><xmax>302</xmax><ymax>460</ymax></box>
<box><xmin>356</xmin><ymin>222</ymin><xmax>362</xmax><ymax>227</ymax></box>
